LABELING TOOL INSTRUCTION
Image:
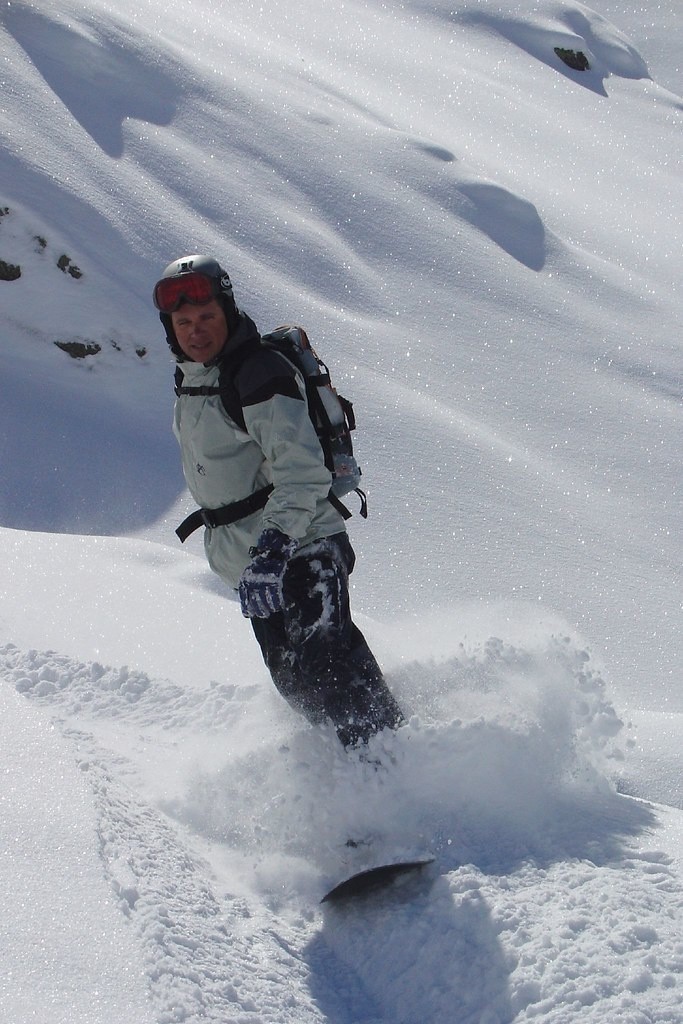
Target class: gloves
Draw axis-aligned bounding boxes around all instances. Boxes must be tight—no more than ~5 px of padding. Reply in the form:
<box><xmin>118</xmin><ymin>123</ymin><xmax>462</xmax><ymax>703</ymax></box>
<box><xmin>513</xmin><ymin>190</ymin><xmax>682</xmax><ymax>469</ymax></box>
<box><xmin>234</xmin><ymin>528</ymin><xmax>298</xmax><ymax>619</ymax></box>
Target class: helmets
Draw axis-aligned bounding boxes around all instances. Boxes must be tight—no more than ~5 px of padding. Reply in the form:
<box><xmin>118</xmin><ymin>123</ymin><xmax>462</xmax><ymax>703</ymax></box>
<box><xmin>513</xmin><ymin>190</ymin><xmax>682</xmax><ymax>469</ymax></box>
<box><xmin>159</xmin><ymin>255</ymin><xmax>234</xmax><ymax>327</ymax></box>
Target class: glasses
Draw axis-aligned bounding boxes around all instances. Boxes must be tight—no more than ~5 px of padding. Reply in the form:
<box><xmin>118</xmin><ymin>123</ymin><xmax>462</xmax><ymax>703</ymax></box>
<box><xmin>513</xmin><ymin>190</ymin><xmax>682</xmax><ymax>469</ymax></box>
<box><xmin>153</xmin><ymin>271</ymin><xmax>218</xmax><ymax>313</ymax></box>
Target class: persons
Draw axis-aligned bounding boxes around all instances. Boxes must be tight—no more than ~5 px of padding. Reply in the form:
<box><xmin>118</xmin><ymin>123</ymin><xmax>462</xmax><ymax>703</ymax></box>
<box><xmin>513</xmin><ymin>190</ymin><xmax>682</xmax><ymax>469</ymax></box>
<box><xmin>153</xmin><ymin>255</ymin><xmax>408</xmax><ymax>765</ymax></box>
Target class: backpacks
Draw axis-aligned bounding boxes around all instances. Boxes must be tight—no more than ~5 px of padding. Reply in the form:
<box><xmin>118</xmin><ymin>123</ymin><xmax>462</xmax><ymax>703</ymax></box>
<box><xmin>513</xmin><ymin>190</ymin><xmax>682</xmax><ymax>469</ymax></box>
<box><xmin>261</xmin><ymin>324</ymin><xmax>362</xmax><ymax>498</ymax></box>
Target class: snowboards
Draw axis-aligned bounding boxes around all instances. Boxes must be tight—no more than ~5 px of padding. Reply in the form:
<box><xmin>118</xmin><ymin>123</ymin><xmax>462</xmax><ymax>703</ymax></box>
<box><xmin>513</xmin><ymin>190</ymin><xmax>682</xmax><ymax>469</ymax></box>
<box><xmin>316</xmin><ymin>854</ymin><xmax>437</xmax><ymax>905</ymax></box>
